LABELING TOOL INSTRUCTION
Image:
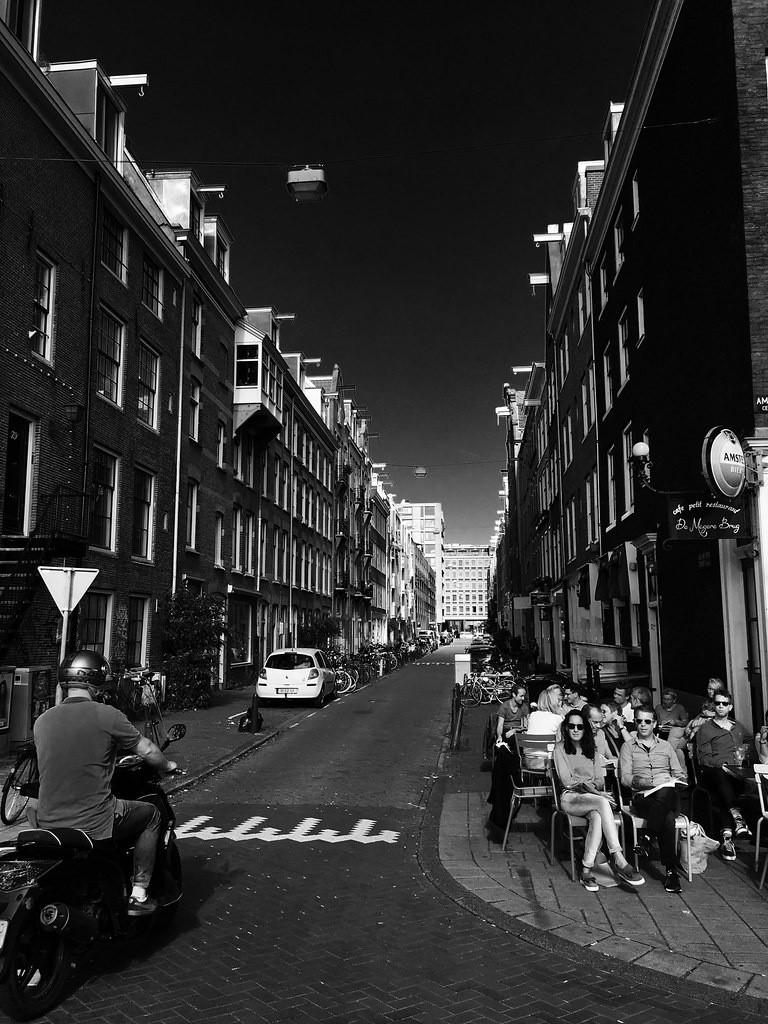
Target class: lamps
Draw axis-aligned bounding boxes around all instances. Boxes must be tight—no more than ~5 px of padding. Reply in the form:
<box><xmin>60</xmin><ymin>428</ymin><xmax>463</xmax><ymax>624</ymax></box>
<box><xmin>48</xmin><ymin>395</ymin><xmax>84</xmax><ymax>438</ymax></box>
<box><xmin>288</xmin><ymin>164</ymin><xmax>331</xmax><ymax>205</ymax></box>
<box><xmin>628</xmin><ymin>442</ymin><xmax>668</xmax><ymax>498</ymax></box>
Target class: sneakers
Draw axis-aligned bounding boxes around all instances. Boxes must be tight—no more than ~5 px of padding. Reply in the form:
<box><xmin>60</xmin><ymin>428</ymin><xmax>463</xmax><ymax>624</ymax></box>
<box><xmin>722</xmin><ymin>838</ymin><xmax>736</xmax><ymax>860</ymax></box>
<box><xmin>632</xmin><ymin>837</ymin><xmax>651</xmax><ymax>856</ymax></box>
<box><xmin>579</xmin><ymin>873</ymin><xmax>599</xmax><ymax>892</ymax></box>
<box><xmin>128</xmin><ymin>895</ymin><xmax>158</xmax><ymax>915</ymax></box>
<box><xmin>665</xmin><ymin>870</ymin><xmax>683</xmax><ymax>892</ymax></box>
<box><xmin>615</xmin><ymin>863</ymin><xmax>645</xmax><ymax>885</ymax></box>
<box><xmin>735</xmin><ymin>816</ymin><xmax>753</xmax><ymax>837</ymax></box>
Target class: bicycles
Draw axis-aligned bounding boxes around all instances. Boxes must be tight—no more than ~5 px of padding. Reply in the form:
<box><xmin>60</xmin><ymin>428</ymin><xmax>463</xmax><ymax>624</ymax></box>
<box><xmin>99</xmin><ymin>665</ymin><xmax>164</xmax><ymax>725</ymax></box>
<box><xmin>320</xmin><ymin>628</ymin><xmax>521</xmax><ymax>708</ymax></box>
<box><xmin>0</xmin><ymin>693</ymin><xmax>55</xmax><ymax>826</ymax></box>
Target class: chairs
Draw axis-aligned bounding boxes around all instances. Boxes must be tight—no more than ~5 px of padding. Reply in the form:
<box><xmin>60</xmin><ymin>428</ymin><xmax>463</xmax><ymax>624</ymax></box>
<box><xmin>490</xmin><ymin>716</ymin><xmax>768</xmax><ymax>890</ymax></box>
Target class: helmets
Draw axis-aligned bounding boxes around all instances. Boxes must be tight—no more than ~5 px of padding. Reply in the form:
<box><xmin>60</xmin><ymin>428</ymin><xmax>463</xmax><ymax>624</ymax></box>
<box><xmin>59</xmin><ymin>650</ymin><xmax>112</xmax><ymax>691</ymax></box>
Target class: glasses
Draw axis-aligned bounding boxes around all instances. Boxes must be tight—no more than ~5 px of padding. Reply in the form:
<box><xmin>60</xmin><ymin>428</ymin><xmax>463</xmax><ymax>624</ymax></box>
<box><xmin>634</xmin><ymin>720</ymin><xmax>655</xmax><ymax>724</ymax></box>
<box><xmin>714</xmin><ymin>701</ymin><xmax>730</xmax><ymax>706</ymax></box>
<box><xmin>568</xmin><ymin>723</ymin><xmax>585</xmax><ymax>730</ymax></box>
<box><xmin>601</xmin><ymin>709</ymin><xmax>612</xmax><ymax>713</ymax></box>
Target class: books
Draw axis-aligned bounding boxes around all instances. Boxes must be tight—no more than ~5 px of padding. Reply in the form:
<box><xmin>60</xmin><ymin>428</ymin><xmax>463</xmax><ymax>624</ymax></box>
<box><xmin>643</xmin><ymin>780</ymin><xmax>688</xmax><ymax>797</ymax></box>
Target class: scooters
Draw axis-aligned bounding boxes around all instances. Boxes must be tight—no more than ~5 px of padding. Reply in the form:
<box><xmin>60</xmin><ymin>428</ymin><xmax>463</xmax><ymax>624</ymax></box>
<box><xmin>0</xmin><ymin>723</ymin><xmax>187</xmax><ymax>1024</ymax></box>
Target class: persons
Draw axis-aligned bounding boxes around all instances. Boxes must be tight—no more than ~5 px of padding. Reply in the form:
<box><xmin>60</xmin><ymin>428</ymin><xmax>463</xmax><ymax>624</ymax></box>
<box><xmin>33</xmin><ymin>651</ymin><xmax>178</xmax><ymax>914</ymax></box>
<box><xmin>531</xmin><ymin>638</ymin><xmax>539</xmax><ymax>668</ymax></box>
<box><xmin>492</xmin><ymin>675</ymin><xmax>768</xmax><ymax>893</ymax></box>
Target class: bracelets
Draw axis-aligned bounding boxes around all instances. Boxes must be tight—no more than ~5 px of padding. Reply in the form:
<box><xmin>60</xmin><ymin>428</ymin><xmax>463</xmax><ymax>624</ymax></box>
<box><xmin>759</xmin><ymin>741</ymin><xmax>766</xmax><ymax>744</ymax></box>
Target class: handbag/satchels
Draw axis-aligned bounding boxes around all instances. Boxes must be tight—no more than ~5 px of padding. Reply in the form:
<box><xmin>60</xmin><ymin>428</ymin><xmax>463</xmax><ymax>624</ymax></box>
<box><xmin>679</xmin><ymin>821</ymin><xmax>720</xmax><ymax>874</ymax></box>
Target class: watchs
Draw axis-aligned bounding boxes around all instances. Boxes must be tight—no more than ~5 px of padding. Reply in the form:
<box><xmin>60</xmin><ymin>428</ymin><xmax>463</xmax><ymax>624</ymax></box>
<box><xmin>620</xmin><ymin>726</ymin><xmax>627</xmax><ymax>730</ymax></box>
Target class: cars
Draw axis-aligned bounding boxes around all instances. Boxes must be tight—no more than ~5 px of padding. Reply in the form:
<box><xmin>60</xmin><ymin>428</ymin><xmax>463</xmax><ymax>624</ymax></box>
<box><xmin>256</xmin><ymin>647</ymin><xmax>340</xmax><ymax>709</ymax></box>
